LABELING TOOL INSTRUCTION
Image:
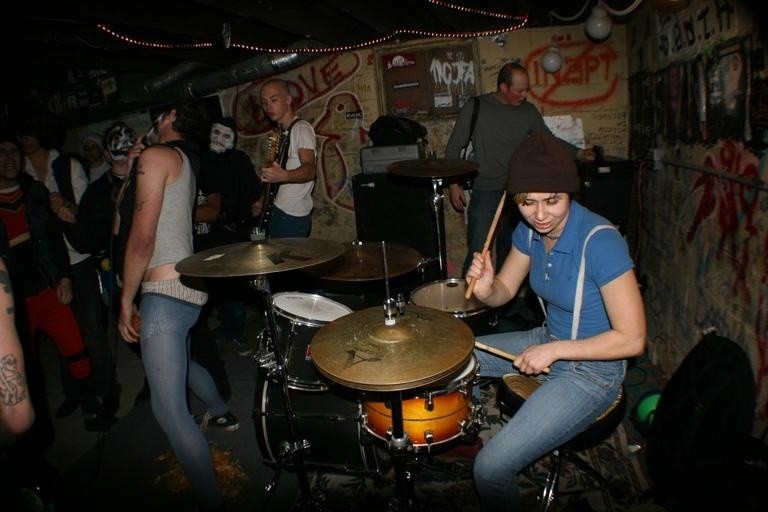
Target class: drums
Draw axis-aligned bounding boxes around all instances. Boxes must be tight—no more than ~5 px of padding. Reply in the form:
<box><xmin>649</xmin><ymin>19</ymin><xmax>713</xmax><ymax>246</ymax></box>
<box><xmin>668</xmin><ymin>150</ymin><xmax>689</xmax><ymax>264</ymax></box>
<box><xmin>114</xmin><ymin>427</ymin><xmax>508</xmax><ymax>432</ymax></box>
<box><xmin>253</xmin><ymin>372</ymin><xmax>396</xmax><ymax>478</ymax></box>
<box><xmin>261</xmin><ymin>292</ymin><xmax>354</xmax><ymax>390</ymax></box>
<box><xmin>362</xmin><ymin>353</ymin><xmax>480</xmax><ymax>447</ymax></box>
<box><xmin>410</xmin><ymin>277</ymin><xmax>491</xmax><ymax>315</ymax></box>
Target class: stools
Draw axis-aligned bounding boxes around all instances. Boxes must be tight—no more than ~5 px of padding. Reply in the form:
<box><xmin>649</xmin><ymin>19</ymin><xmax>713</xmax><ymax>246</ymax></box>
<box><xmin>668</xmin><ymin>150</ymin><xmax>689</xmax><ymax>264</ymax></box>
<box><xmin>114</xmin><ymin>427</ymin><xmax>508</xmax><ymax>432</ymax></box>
<box><xmin>497</xmin><ymin>373</ymin><xmax>651</xmax><ymax>512</ymax></box>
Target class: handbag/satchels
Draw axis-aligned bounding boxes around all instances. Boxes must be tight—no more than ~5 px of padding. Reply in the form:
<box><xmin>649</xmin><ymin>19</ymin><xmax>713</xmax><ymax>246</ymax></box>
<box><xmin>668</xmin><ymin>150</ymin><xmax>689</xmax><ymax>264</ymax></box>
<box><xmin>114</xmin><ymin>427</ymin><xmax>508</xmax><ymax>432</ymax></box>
<box><xmin>368</xmin><ymin>115</ymin><xmax>429</xmax><ymax>146</ymax></box>
<box><xmin>444</xmin><ymin>96</ymin><xmax>480</xmax><ymax>190</ymax></box>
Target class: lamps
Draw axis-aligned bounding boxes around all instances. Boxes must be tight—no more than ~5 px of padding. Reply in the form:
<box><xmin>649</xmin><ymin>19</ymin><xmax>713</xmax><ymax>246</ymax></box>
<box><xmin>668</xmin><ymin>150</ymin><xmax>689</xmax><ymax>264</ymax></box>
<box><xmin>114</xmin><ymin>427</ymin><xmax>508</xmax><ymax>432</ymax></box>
<box><xmin>585</xmin><ymin>0</ymin><xmax>613</xmax><ymax>39</ymax></box>
<box><xmin>541</xmin><ymin>39</ymin><xmax>563</xmax><ymax>72</ymax></box>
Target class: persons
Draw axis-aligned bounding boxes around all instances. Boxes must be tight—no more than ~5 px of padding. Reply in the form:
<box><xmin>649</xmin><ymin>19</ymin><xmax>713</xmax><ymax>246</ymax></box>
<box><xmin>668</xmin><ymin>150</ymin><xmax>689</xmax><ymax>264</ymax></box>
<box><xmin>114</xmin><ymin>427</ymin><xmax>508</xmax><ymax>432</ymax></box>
<box><xmin>193</xmin><ymin>117</ymin><xmax>260</xmax><ymax>347</ymax></box>
<box><xmin>0</xmin><ymin>140</ymin><xmax>111</xmax><ymax>430</ymax></box>
<box><xmin>77</xmin><ymin>120</ymin><xmax>143</xmax><ymax>264</ymax></box>
<box><xmin>115</xmin><ymin>99</ymin><xmax>242</xmax><ymax>510</ymax></box>
<box><xmin>81</xmin><ymin>130</ymin><xmax>112</xmax><ymax>194</ymax></box>
<box><xmin>0</xmin><ymin>264</ymin><xmax>37</xmax><ymax>434</ymax></box>
<box><xmin>477</xmin><ymin>130</ymin><xmax>645</xmax><ymax>512</ymax></box>
<box><xmin>238</xmin><ymin>79</ymin><xmax>317</xmax><ymax>365</ymax></box>
<box><xmin>16</xmin><ymin>114</ymin><xmax>123</xmax><ymax>414</ymax></box>
<box><xmin>445</xmin><ymin>61</ymin><xmax>595</xmax><ymax>280</ymax></box>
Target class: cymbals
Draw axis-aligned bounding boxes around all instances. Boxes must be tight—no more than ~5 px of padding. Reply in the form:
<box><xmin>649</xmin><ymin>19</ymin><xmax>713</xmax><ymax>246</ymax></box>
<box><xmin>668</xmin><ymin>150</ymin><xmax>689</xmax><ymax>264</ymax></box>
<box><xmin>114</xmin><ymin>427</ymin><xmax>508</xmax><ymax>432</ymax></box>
<box><xmin>310</xmin><ymin>305</ymin><xmax>476</xmax><ymax>390</ymax></box>
<box><xmin>387</xmin><ymin>159</ymin><xmax>480</xmax><ymax>178</ymax></box>
<box><xmin>299</xmin><ymin>243</ymin><xmax>422</xmax><ymax>282</ymax></box>
<box><xmin>174</xmin><ymin>236</ymin><xmax>345</xmax><ymax>278</ymax></box>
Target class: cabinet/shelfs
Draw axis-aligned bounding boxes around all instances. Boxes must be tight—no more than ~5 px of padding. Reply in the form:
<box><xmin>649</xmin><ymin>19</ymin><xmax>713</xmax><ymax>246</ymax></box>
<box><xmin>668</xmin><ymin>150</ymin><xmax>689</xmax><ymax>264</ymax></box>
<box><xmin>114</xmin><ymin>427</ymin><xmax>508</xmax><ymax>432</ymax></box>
<box><xmin>353</xmin><ymin>172</ymin><xmax>446</xmax><ymax>285</ymax></box>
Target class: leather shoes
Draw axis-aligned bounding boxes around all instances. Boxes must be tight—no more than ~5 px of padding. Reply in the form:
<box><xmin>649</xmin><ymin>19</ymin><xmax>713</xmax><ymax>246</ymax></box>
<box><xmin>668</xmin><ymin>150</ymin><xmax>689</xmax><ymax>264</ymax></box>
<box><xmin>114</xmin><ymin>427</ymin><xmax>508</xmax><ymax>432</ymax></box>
<box><xmin>83</xmin><ymin>400</ymin><xmax>101</xmax><ymax>419</ymax></box>
<box><xmin>57</xmin><ymin>395</ymin><xmax>78</xmax><ymax>417</ymax></box>
<box><xmin>138</xmin><ymin>387</ymin><xmax>152</xmax><ymax>398</ymax></box>
<box><xmin>210</xmin><ymin>364</ymin><xmax>232</xmax><ymax>403</ymax></box>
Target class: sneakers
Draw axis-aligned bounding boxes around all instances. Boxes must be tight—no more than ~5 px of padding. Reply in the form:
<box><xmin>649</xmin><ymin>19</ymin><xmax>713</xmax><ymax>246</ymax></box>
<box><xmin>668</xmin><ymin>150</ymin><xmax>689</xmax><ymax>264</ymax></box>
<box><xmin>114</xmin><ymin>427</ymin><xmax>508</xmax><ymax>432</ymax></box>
<box><xmin>190</xmin><ymin>411</ymin><xmax>240</xmax><ymax>433</ymax></box>
<box><xmin>231</xmin><ymin>335</ymin><xmax>253</xmax><ymax>358</ymax></box>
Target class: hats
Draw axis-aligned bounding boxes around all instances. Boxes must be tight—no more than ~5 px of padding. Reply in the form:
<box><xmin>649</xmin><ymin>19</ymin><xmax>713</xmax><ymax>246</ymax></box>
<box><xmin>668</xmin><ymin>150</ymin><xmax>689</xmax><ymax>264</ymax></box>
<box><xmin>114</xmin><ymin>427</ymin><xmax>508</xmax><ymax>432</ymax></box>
<box><xmin>508</xmin><ymin>133</ymin><xmax>582</xmax><ymax>194</ymax></box>
<box><xmin>80</xmin><ymin>135</ymin><xmax>107</xmax><ymax>155</ymax></box>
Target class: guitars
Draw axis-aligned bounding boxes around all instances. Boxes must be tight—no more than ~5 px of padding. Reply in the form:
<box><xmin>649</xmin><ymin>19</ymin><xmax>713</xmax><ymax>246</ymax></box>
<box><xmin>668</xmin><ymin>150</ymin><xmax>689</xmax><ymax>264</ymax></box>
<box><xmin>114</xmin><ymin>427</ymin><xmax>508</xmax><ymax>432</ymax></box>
<box><xmin>258</xmin><ymin>132</ymin><xmax>280</xmax><ymax>227</ymax></box>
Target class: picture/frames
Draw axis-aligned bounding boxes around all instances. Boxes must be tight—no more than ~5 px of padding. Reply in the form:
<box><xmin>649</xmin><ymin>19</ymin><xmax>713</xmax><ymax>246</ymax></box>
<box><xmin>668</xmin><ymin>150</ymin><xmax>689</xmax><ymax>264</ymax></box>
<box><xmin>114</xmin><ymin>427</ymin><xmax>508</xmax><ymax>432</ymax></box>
<box><xmin>373</xmin><ymin>38</ymin><xmax>480</xmax><ymax>121</ymax></box>
